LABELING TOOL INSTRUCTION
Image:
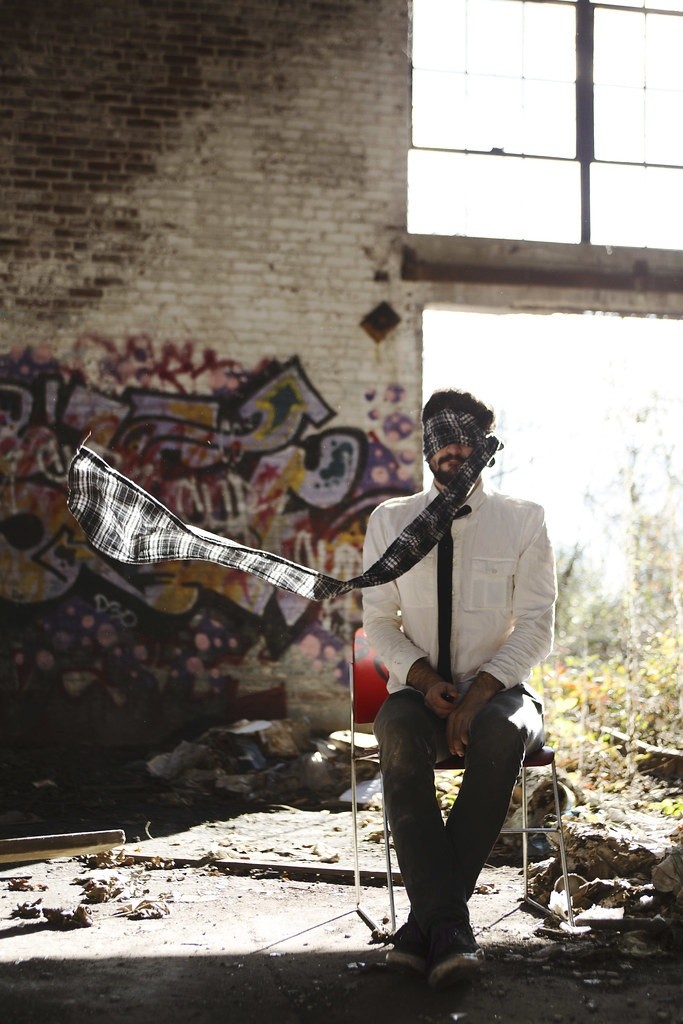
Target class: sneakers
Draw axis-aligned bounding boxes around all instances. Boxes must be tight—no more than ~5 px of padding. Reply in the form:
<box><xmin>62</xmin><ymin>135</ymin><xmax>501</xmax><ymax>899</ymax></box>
<box><xmin>425</xmin><ymin>920</ymin><xmax>483</xmax><ymax>987</ymax></box>
<box><xmin>385</xmin><ymin>922</ymin><xmax>429</xmax><ymax>971</ymax></box>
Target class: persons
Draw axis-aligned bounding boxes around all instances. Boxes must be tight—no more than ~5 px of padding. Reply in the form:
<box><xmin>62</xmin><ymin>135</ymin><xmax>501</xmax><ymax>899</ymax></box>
<box><xmin>359</xmin><ymin>391</ymin><xmax>558</xmax><ymax>983</ymax></box>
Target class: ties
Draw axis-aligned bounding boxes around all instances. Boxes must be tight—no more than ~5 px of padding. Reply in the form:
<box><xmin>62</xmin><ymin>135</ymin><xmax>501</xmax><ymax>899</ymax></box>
<box><xmin>436</xmin><ymin>506</ymin><xmax>472</xmax><ymax>701</ymax></box>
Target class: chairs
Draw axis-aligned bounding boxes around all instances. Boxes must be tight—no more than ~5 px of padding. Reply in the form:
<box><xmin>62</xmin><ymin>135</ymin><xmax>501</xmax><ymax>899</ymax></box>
<box><xmin>352</xmin><ymin>625</ymin><xmax>581</xmax><ymax>944</ymax></box>
